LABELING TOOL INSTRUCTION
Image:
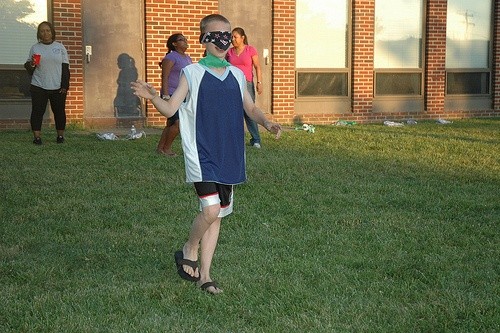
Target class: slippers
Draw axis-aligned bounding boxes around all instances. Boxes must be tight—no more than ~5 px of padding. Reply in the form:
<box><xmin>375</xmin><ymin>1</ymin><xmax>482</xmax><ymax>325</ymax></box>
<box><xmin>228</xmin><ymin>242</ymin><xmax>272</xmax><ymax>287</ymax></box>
<box><xmin>195</xmin><ymin>280</ymin><xmax>217</xmax><ymax>295</ymax></box>
<box><xmin>174</xmin><ymin>251</ymin><xmax>199</xmax><ymax>282</ymax></box>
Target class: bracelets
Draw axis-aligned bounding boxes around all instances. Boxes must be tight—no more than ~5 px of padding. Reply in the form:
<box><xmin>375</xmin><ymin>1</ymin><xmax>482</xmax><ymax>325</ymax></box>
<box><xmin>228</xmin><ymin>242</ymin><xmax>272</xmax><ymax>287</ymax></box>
<box><xmin>257</xmin><ymin>81</ymin><xmax>262</xmax><ymax>84</ymax></box>
<box><xmin>162</xmin><ymin>94</ymin><xmax>170</xmax><ymax>100</ymax></box>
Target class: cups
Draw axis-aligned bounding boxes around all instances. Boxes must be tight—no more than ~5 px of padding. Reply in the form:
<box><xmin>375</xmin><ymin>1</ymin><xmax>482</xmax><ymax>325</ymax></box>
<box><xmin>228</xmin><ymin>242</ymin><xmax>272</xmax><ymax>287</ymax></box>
<box><xmin>33</xmin><ymin>54</ymin><xmax>41</xmax><ymax>64</ymax></box>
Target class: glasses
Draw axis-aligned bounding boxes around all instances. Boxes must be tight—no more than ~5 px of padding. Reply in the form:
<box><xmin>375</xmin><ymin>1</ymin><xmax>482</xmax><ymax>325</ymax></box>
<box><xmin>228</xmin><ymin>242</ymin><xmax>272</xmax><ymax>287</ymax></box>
<box><xmin>176</xmin><ymin>38</ymin><xmax>189</xmax><ymax>41</ymax></box>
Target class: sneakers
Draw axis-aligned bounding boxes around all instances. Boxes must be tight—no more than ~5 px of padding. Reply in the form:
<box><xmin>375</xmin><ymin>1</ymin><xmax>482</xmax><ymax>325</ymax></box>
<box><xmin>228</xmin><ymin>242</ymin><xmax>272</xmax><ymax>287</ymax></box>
<box><xmin>33</xmin><ymin>137</ymin><xmax>42</xmax><ymax>145</ymax></box>
<box><xmin>57</xmin><ymin>136</ymin><xmax>64</xmax><ymax>144</ymax></box>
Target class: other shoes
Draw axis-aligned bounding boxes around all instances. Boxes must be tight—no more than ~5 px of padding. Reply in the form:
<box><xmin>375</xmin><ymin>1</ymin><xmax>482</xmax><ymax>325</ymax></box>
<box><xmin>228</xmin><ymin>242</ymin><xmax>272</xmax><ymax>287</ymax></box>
<box><xmin>302</xmin><ymin>124</ymin><xmax>309</xmax><ymax>130</ymax></box>
<box><xmin>309</xmin><ymin>125</ymin><xmax>315</xmax><ymax>133</ymax></box>
<box><xmin>253</xmin><ymin>142</ymin><xmax>260</xmax><ymax>149</ymax></box>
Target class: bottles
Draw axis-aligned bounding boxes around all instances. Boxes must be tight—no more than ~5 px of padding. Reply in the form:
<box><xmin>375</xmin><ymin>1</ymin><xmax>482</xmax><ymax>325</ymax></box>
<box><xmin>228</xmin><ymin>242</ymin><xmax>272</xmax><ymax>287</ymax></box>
<box><xmin>130</xmin><ymin>125</ymin><xmax>136</xmax><ymax>134</ymax></box>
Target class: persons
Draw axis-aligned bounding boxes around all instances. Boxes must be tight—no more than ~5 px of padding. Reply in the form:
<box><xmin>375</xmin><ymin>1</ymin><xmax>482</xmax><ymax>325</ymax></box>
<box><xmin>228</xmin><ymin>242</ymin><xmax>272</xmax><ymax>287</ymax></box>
<box><xmin>24</xmin><ymin>22</ymin><xmax>71</xmax><ymax>145</ymax></box>
<box><xmin>130</xmin><ymin>14</ymin><xmax>282</xmax><ymax>294</ymax></box>
<box><xmin>225</xmin><ymin>27</ymin><xmax>263</xmax><ymax>148</ymax></box>
<box><xmin>156</xmin><ymin>33</ymin><xmax>193</xmax><ymax>156</ymax></box>
